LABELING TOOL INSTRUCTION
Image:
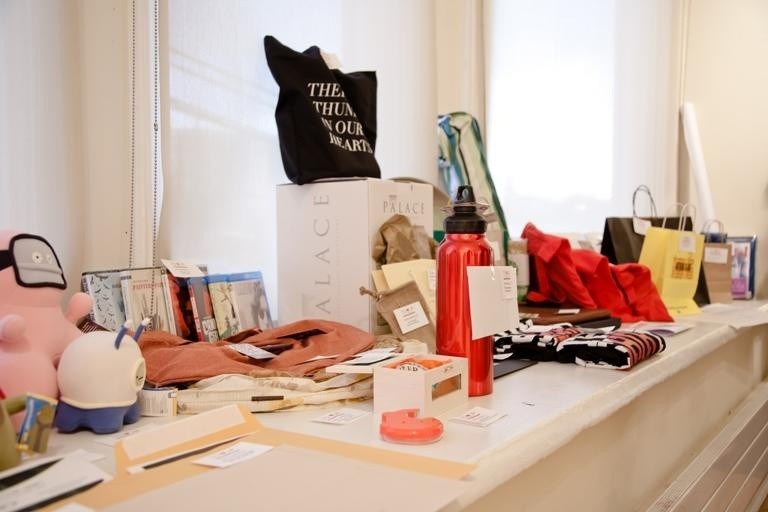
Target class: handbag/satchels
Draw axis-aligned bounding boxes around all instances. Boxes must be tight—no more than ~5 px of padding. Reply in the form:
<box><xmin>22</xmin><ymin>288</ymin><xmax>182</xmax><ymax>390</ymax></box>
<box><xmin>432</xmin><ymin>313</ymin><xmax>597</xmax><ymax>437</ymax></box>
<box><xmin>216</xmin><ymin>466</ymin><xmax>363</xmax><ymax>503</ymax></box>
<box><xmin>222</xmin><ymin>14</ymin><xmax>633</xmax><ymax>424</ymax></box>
<box><xmin>600</xmin><ymin>184</ymin><xmax>711</xmax><ymax>307</ymax></box>
<box><xmin>262</xmin><ymin>34</ymin><xmax>382</xmax><ymax>186</ymax></box>
<box><xmin>636</xmin><ymin>199</ymin><xmax>705</xmax><ymax>318</ymax></box>
<box><xmin>698</xmin><ymin>218</ymin><xmax>735</xmax><ymax>304</ymax></box>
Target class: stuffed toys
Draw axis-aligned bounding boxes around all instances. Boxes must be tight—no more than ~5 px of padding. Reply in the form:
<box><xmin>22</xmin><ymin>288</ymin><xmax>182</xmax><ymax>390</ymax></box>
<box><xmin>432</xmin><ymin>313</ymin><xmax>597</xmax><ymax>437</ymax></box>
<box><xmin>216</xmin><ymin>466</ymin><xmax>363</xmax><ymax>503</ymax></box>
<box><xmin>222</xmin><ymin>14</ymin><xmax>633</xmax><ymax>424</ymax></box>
<box><xmin>0</xmin><ymin>228</ymin><xmax>93</xmax><ymax>434</ymax></box>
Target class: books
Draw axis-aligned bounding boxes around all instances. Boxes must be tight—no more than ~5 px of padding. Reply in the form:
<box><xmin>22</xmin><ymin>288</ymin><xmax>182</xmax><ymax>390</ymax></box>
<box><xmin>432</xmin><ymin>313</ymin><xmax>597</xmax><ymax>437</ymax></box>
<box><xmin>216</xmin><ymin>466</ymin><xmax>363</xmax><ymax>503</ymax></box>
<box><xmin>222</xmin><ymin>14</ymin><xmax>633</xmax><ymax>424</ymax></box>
<box><xmin>326</xmin><ymin>351</ymin><xmax>414</xmax><ymax>373</ymax></box>
<box><xmin>176</xmin><ymin>384</ymin><xmax>264</xmax><ymax>400</ymax></box>
<box><xmin>119</xmin><ymin>266</ymin><xmax>171</xmax><ymax>338</ymax></box>
<box><xmin>161</xmin><ymin>264</ymin><xmax>210</xmax><ymax>345</ymax></box>
<box><xmin>177</xmin><ymin>395</ymin><xmax>304</xmax><ymax>414</ymax></box>
<box><xmin>185</xmin><ymin>276</ymin><xmax>219</xmax><ymax>346</ymax></box>
<box><xmin>226</xmin><ymin>271</ymin><xmax>274</xmax><ymax>335</ymax></box>
<box><xmin>205</xmin><ymin>273</ymin><xmax>240</xmax><ymax>341</ymax></box>
<box><xmin>78</xmin><ymin>269</ymin><xmax>125</xmax><ymax>335</ymax></box>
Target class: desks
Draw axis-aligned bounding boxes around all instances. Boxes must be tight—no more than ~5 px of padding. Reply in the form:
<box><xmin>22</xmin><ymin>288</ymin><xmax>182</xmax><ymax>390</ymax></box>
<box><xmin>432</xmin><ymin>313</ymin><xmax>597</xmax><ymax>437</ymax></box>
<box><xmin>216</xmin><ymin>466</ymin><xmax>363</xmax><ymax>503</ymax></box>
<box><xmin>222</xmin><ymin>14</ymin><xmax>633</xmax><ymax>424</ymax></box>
<box><xmin>1</xmin><ymin>291</ymin><xmax>768</xmax><ymax>512</ymax></box>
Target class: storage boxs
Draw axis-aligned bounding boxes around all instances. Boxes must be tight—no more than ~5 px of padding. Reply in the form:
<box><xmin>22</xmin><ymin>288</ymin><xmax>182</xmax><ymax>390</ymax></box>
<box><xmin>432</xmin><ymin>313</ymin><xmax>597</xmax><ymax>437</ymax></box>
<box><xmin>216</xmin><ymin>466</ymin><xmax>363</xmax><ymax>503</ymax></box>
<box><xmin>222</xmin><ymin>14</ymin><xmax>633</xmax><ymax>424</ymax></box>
<box><xmin>270</xmin><ymin>176</ymin><xmax>435</xmax><ymax>337</ymax></box>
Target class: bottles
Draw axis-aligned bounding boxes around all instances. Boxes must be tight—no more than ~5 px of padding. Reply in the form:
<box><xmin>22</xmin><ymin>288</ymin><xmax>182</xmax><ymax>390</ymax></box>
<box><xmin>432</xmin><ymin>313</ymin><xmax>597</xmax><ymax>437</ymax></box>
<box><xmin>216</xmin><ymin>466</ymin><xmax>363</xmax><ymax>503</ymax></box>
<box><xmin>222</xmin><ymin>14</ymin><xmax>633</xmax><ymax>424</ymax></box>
<box><xmin>436</xmin><ymin>184</ymin><xmax>494</xmax><ymax>398</ymax></box>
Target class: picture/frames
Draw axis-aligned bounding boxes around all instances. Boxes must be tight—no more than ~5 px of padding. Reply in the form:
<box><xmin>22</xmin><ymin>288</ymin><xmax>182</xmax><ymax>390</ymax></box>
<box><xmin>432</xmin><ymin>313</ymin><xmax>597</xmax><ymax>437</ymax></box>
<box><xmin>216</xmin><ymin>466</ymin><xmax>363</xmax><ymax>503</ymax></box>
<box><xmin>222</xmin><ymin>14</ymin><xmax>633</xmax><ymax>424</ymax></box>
<box><xmin>726</xmin><ymin>236</ymin><xmax>757</xmax><ymax>300</ymax></box>
